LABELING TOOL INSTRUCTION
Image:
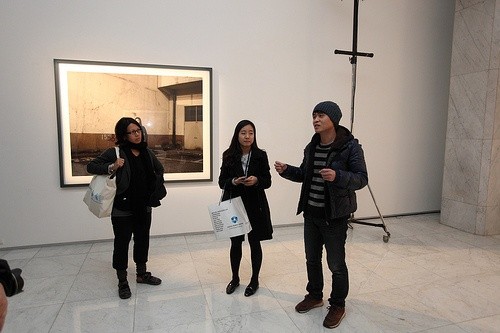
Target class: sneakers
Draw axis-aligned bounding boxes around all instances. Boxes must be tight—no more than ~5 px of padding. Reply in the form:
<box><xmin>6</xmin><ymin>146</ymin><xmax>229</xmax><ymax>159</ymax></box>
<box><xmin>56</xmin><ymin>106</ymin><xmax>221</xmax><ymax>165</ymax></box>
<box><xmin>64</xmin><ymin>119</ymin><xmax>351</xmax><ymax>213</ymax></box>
<box><xmin>295</xmin><ymin>295</ymin><xmax>324</xmax><ymax>313</ymax></box>
<box><xmin>323</xmin><ymin>304</ymin><xmax>346</xmax><ymax>328</ymax></box>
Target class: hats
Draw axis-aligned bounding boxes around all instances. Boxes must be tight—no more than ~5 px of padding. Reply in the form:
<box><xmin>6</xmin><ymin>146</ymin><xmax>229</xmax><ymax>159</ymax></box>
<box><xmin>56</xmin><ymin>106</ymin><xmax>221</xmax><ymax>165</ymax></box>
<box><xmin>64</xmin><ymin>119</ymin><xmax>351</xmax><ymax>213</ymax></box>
<box><xmin>313</xmin><ymin>101</ymin><xmax>342</xmax><ymax>126</ymax></box>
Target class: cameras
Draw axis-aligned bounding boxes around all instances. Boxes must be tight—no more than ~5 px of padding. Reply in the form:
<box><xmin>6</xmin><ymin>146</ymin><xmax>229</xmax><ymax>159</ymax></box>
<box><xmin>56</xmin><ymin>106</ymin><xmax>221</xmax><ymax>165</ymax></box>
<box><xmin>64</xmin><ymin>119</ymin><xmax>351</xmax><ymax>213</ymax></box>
<box><xmin>0</xmin><ymin>259</ymin><xmax>24</xmax><ymax>297</ymax></box>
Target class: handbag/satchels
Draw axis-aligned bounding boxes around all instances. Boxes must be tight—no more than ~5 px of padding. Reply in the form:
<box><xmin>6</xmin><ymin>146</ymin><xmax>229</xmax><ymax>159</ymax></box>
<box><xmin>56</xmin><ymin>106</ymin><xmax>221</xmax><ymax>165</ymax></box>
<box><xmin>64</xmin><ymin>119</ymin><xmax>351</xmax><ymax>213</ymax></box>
<box><xmin>156</xmin><ymin>174</ymin><xmax>167</xmax><ymax>200</ymax></box>
<box><xmin>82</xmin><ymin>147</ymin><xmax>120</xmax><ymax>218</ymax></box>
<box><xmin>208</xmin><ymin>187</ymin><xmax>252</xmax><ymax>239</ymax></box>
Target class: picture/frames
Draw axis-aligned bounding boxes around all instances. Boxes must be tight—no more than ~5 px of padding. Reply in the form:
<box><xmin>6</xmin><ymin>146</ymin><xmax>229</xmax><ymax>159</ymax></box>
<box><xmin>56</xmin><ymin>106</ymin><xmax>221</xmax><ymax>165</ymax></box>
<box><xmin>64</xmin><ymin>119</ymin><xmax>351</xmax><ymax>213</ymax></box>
<box><xmin>54</xmin><ymin>59</ymin><xmax>213</xmax><ymax>187</ymax></box>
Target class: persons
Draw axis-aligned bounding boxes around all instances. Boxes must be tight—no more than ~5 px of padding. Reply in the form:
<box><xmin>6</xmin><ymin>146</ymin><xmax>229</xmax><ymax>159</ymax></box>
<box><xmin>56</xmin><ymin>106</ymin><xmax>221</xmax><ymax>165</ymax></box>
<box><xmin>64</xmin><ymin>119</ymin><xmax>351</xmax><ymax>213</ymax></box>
<box><xmin>274</xmin><ymin>101</ymin><xmax>368</xmax><ymax>328</ymax></box>
<box><xmin>0</xmin><ymin>283</ymin><xmax>9</xmax><ymax>333</ymax></box>
<box><xmin>218</xmin><ymin>120</ymin><xmax>274</xmax><ymax>297</ymax></box>
<box><xmin>87</xmin><ymin>117</ymin><xmax>168</xmax><ymax>299</ymax></box>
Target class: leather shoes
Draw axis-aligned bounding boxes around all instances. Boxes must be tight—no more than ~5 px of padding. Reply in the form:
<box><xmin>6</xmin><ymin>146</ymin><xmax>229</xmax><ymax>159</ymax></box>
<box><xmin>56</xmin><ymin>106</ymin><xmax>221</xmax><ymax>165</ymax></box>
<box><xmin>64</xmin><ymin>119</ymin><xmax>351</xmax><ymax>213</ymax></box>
<box><xmin>226</xmin><ymin>280</ymin><xmax>239</xmax><ymax>294</ymax></box>
<box><xmin>118</xmin><ymin>279</ymin><xmax>131</xmax><ymax>300</ymax></box>
<box><xmin>245</xmin><ymin>282</ymin><xmax>259</xmax><ymax>296</ymax></box>
<box><xmin>136</xmin><ymin>272</ymin><xmax>161</xmax><ymax>285</ymax></box>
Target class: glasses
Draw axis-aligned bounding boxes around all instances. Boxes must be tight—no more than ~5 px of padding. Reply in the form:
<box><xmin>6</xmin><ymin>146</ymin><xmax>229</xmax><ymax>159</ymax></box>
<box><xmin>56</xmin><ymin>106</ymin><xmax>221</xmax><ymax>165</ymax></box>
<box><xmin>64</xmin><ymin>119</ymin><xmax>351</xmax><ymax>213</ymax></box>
<box><xmin>128</xmin><ymin>128</ymin><xmax>141</xmax><ymax>135</ymax></box>
